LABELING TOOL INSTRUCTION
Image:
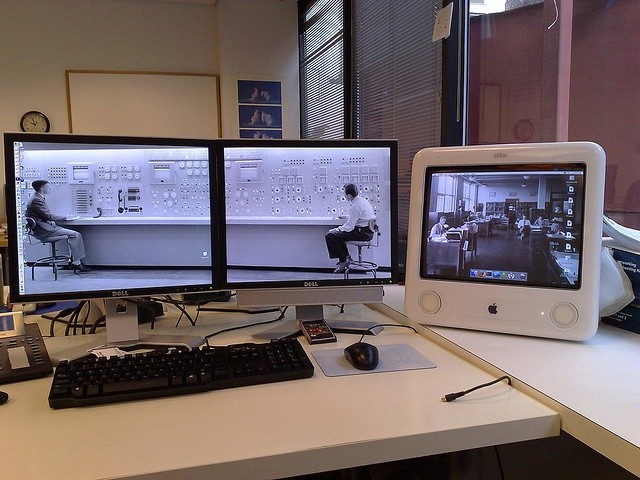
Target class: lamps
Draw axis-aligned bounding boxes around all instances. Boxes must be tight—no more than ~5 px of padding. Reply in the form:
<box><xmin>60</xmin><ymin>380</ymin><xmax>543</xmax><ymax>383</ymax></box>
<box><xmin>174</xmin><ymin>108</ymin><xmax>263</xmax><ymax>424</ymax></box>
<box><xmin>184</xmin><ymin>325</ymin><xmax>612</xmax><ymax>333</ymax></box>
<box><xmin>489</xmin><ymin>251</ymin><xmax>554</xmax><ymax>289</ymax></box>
<box><xmin>522</xmin><ymin>183</ymin><xmax>526</xmax><ymax>187</ymax></box>
<box><xmin>469</xmin><ymin>176</ymin><xmax>476</xmax><ymax>181</ymax></box>
<box><xmin>523</xmin><ymin>176</ymin><xmax>530</xmax><ymax>179</ymax></box>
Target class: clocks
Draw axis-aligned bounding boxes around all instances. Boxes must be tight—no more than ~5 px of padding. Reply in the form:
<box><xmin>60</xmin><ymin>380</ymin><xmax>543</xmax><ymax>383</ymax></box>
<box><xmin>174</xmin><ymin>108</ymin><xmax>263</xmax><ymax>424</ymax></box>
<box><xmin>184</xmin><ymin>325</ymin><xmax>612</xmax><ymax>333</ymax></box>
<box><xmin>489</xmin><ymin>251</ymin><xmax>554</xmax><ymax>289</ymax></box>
<box><xmin>20</xmin><ymin>110</ymin><xmax>50</xmax><ymax>133</ymax></box>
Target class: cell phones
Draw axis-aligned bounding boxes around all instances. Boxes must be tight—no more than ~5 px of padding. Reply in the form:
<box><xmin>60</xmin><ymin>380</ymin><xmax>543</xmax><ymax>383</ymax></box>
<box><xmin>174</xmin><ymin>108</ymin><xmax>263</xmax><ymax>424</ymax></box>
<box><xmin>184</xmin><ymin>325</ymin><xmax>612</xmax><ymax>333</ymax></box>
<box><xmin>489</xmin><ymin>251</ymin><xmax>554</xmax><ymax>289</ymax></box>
<box><xmin>300</xmin><ymin>319</ymin><xmax>338</xmax><ymax>345</ymax></box>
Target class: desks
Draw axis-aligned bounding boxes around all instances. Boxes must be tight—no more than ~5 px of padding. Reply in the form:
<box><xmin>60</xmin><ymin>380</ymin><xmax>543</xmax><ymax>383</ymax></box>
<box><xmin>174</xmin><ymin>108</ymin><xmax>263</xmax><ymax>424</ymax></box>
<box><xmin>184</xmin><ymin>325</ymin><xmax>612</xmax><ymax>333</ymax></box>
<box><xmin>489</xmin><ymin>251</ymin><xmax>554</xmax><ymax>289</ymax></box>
<box><xmin>527</xmin><ymin>225</ymin><xmax>566</xmax><ymax>257</ymax></box>
<box><xmin>1</xmin><ymin>295</ymin><xmax>560</xmax><ymax>479</ymax></box>
<box><xmin>464</xmin><ymin>218</ymin><xmax>492</xmax><ymax>237</ymax></box>
<box><xmin>543</xmin><ymin>248</ymin><xmax>579</xmax><ymax>285</ymax></box>
<box><xmin>371</xmin><ymin>282</ymin><xmax>639</xmax><ymax>476</ymax></box>
<box><xmin>426</xmin><ymin>236</ymin><xmax>466</xmax><ymax>277</ymax></box>
<box><xmin>466</xmin><ymin>231</ymin><xmax>478</xmax><ymax>261</ymax></box>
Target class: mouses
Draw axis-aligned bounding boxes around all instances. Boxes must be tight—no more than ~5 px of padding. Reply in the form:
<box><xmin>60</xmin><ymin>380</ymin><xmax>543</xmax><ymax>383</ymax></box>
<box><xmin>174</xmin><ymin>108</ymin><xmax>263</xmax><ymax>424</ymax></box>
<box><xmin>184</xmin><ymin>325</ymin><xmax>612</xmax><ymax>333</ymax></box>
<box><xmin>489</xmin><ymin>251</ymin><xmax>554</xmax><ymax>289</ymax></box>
<box><xmin>346</xmin><ymin>342</ymin><xmax>379</xmax><ymax>371</ymax></box>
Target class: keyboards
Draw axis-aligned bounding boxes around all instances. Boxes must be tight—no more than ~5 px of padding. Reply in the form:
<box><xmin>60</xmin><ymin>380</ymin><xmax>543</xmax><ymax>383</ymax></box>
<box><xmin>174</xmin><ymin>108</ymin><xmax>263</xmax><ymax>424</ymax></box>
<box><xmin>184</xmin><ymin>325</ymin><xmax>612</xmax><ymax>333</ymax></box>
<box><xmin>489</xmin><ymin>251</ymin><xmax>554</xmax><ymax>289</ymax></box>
<box><xmin>49</xmin><ymin>336</ymin><xmax>315</xmax><ymax>410</ymax></box>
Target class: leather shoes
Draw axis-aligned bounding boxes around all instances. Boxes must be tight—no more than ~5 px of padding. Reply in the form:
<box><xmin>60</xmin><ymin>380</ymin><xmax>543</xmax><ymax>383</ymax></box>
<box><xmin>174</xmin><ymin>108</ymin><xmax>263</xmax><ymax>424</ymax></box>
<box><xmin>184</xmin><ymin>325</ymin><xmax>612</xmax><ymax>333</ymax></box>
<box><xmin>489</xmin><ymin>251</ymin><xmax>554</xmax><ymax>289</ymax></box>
<box><xmin>67</xmin><ymin>261</ymin><xmax>71</xmax><ymax>266</ymax></box>
<box><xmin>334</xmin><ymin>259</ymin><xmax>350</xmax><ymax>274</ymax></box>
<box><xmin>72</xmin><ymin>263</ymin><xmax>88</xmax><ymax>272</ymax></box>
<box><xmin>336</xmin><ymin>255</ymin><xmax>352</xmax><ymax>265</ymax></box>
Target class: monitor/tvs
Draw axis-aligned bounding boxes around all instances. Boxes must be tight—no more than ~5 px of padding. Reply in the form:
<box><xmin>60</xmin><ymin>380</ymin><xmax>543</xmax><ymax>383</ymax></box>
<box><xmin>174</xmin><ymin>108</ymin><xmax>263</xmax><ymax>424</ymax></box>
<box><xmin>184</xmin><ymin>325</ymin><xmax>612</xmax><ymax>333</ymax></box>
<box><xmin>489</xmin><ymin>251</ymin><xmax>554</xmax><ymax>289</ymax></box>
<box><xmin>2</xmin><ymin>133</ymin><xmax>217</xmax><ymax>365</ymax></box>
<box><xmin>405</xmin><ymin>142</ymin><xmax>607</xmax><ymax>342</ymax></box>
<box><xmin>216</xmin><ymin>138</ymin><xmax>400</xmax><ymax>346</ymax></box>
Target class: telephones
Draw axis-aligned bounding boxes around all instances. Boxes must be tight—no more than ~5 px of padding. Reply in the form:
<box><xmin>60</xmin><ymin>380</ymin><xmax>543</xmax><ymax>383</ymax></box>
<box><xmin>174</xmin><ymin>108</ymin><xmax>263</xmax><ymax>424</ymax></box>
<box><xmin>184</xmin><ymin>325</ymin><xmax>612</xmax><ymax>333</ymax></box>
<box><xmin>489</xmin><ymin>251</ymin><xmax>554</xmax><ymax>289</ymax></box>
<box><xmin>0</xmin><ymin>311</ymin><xmax>53</xmax><ymax>404</ymax></box>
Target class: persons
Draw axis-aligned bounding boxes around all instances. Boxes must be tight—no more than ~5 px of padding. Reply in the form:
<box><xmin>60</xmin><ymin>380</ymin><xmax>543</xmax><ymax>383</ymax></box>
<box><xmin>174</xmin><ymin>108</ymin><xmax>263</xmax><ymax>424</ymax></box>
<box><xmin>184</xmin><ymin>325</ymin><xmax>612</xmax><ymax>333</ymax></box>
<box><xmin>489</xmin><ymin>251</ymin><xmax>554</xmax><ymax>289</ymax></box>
<box><xmin>25</xmin><ymin>180</ymin><xmax>91</xmax><ymax>272</ymax></box>
<box><xmin>241</xmin><ymin>85</ymin><xmax>259</xmax><ymax>103</ymax></box>
<box><xmin>260</xmin><ymin>87</ymin><xmax>277</xmax><ymax>103</ymax></box>
<box><xmin>517</xmin><ymin>215</ymin><xmax>531</xmax><ymax>241</ymax></box>
<box><xmin>251</xmin><ymin>131</ymin><xmax>261</xmax><ymax>139</ymax></box>
<box><xmin>431</xmin><ymin>216</ymin><xmax>448</xmax><ymax>237</ymax></box>
<box><xmin>261</xmin><ymin>108</ymin><xmax>278</xmax><ymax>127</ymax></box>
<box><xmin>533</xmin><ymin>215</ymin><xmax>547</xmax><ymax>226</ymax></box>
<box><xmin>262</xmin><ymin>132</ymin><xmax>275</xmax><ymax>139</ymax></box>
<box><xmin>455</xmin><ymin>206</ymin><xmax>463</xmax><ymax>226</ymax></box>
<box><xmin>324</xmin><ymin>183</ymin><xmax>377</xmax><ymax>274</ymax></box>
<box><xmin>241</xmin><ymin>108</ymin><xmax>260</xmax><ymax>128</ymax></box>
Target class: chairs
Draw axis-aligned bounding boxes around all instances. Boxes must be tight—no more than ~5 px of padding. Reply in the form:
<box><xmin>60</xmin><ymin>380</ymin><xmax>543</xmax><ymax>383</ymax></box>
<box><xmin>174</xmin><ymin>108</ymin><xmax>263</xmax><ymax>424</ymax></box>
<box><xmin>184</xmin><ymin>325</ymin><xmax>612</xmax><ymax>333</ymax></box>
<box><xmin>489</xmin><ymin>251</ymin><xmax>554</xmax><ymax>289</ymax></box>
<box><xmin>24</xmin><ymin>217</ymin><xmax>76</xmax><ymax>281</ymax></box>
<box><xmin>343</xmin><ymin>219</ymin><xmax>379</xmax><ymax>279</ymax></box>
